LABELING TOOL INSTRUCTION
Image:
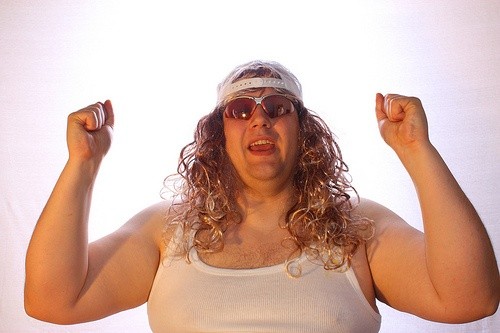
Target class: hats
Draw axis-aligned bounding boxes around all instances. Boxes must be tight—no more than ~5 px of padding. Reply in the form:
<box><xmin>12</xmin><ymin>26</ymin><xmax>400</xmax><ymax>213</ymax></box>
<box><xmin>217</xmin><ymin>59</ymin><xmax>302</xmax><ymax>106</ymax></box>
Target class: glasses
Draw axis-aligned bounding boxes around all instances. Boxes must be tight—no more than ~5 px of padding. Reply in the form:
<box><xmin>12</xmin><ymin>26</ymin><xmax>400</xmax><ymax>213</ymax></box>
<box><xmin>219</xmin><ymin>94</ymin><xmax>299</xmax><ymax>120</ymax></box>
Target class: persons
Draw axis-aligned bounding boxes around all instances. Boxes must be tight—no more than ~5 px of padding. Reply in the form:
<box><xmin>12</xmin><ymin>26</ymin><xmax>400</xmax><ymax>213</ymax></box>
<box><xmin>24</xmin><ymin>59</ymin><xmax>500</xmax><ymax>333</ymax></box>
